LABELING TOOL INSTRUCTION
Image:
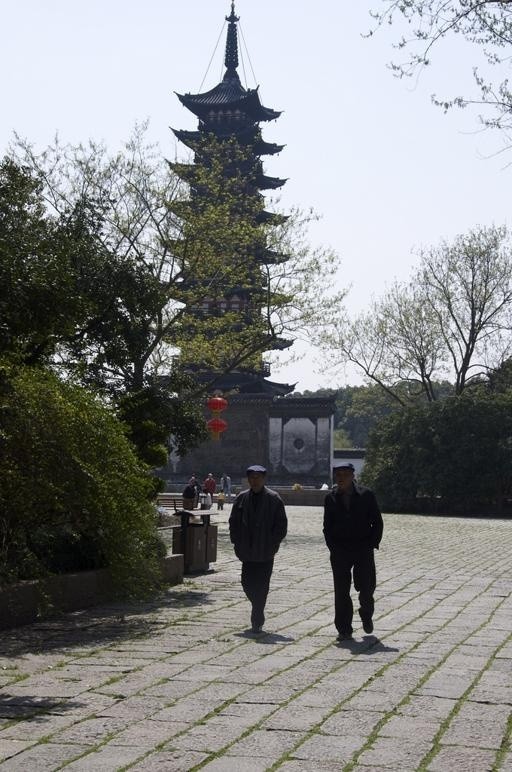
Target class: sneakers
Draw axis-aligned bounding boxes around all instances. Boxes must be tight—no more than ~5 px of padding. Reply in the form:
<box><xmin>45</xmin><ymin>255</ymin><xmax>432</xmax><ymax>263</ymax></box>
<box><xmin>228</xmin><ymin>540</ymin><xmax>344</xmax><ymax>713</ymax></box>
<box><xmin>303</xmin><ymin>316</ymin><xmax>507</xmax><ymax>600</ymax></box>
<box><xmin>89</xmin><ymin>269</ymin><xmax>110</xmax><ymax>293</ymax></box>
<box><xmin>251</xmin><ymin>619</ymin><xmax>264</xmax><ymax>633</ymax></box>
<box><xmin>358</xmin><ymin>607</ymin><xmax>373</xmax><ymax>634</ymax></box>
<box><xmin>335</xmin><ymin>632</ymin><xmax>353</xmax><ymax>642</ymax></box>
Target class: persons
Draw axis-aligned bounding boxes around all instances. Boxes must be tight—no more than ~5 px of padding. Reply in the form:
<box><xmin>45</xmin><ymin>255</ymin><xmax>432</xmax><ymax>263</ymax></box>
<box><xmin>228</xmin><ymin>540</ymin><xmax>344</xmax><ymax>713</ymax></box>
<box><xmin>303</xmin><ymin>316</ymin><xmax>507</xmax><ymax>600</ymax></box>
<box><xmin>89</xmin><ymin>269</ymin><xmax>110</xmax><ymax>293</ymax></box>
<box><xmin>323</xmin><ymin>463</ymin><xmax>384</xmax><ymax>640</ymax></box>
<box><xmin>229</xmin><ymin>465</ymin><xmax>288</xmax><ymax>633</ymax></box>
<box><xmin>182</xmin><ymin>471</ymin><xmax>232</xmax><ymax>510</ymax></box>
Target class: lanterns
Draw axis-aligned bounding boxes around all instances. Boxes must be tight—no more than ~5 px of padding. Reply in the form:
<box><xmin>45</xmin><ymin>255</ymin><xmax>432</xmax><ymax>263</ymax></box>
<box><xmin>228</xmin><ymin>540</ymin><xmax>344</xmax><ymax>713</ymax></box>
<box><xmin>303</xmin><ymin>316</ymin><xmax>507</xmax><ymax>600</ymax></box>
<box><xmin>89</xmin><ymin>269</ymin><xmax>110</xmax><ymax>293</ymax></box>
<box><xmin>206</xmin><ymin>416</ymin><xmax>228</xmax><ymax>441</ymax></box>
<box><xmin>209</xmin><ymin>394</ymin><xmax>229</xmax><ymax>418</ymax></box>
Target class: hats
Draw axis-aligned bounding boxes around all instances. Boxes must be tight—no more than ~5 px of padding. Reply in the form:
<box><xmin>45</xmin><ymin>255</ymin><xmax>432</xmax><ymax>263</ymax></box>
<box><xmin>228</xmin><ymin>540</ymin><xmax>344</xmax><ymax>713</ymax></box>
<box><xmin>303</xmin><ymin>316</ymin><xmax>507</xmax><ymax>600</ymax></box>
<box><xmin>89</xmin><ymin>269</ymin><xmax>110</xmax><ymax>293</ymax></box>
<box><xmin>333</xmin><ymin>462</ymin><xmax>355</xmax><ymax>471</ymax></box>
<box><xmin>246</xmin><ymin>464</ymin><xmax>268</xmax><ymax>475</ymax></box>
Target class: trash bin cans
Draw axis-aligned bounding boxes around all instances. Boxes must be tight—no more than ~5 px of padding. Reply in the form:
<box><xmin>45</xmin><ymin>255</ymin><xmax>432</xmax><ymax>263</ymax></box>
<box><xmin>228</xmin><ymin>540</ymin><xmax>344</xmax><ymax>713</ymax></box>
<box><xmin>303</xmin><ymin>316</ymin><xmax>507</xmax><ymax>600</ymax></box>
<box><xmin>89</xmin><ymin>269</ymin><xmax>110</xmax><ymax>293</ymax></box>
<box><xmin>173</xmin><ymin>510</ymin><xmax>219</xmax><ymax>575</ymax></box>
